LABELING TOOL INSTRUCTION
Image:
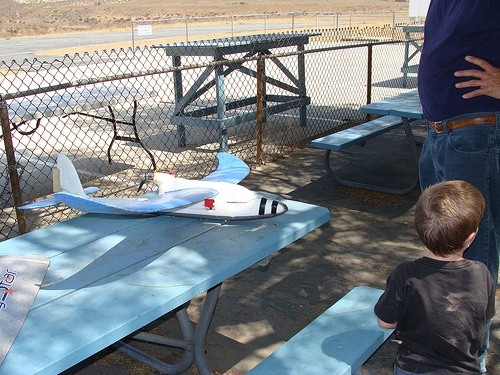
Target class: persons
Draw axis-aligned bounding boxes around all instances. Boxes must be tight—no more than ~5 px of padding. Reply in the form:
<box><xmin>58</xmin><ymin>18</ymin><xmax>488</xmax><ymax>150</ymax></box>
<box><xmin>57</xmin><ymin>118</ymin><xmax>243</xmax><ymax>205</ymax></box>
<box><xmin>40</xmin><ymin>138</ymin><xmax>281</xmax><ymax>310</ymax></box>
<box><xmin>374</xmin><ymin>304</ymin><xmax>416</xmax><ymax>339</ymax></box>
<box><xmin>416</xmin><ymin>0</ymin><xmax>500</xmax><ymax>375</ymax></box>
<box><xmin>374</xmin><ymin>180</ymin><xmax>496</xmax><ymax>375</ymax></box>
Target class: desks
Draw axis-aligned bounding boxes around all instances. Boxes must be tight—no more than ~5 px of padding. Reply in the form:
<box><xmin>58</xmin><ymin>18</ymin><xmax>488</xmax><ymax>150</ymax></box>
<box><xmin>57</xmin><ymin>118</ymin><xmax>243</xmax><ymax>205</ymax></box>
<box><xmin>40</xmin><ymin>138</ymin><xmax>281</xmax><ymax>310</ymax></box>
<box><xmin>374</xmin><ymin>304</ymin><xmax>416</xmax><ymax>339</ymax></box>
<box><xmin>0</xmin><ymin>87</ymin><xmax>158</xmax><ymax>170</ymax></box>
<box><xmin>0</xmin><ymin>199</ymin><xmax>329</xmax><ymax>375</ymax></box>
<box><xmin>401</xmin><ymin>25</ymin><xmax>425</xmax><ymax>88</ymax></box>
<box><xmin>360</xmin><ymin>89</ymin><xmax>426</xmax><ymax>195</ymax></box>
<box><xmin>151</xmin><ymin>33</ymin><xmax>322</xmax><ymax>152</ymax></box>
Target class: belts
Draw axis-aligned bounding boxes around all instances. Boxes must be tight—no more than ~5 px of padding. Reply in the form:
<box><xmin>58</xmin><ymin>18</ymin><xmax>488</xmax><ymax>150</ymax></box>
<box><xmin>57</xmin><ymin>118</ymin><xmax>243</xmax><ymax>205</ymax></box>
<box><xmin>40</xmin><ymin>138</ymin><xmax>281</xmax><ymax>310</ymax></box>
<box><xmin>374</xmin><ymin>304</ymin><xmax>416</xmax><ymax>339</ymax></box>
<box><xmin>428</xmin><ymin>116</ymin><xmax>496</xmax><ymax>133</ymax></box>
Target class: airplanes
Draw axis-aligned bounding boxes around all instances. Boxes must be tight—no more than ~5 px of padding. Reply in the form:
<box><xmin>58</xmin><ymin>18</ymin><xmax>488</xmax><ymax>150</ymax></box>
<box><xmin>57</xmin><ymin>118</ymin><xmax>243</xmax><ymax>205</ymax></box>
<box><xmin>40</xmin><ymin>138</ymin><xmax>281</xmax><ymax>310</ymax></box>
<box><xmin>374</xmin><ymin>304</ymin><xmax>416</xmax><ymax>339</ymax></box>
<box><xmin>17</xmin><ymin>150</ymin><xmax>288</xmax><ymax>222</ymax></box>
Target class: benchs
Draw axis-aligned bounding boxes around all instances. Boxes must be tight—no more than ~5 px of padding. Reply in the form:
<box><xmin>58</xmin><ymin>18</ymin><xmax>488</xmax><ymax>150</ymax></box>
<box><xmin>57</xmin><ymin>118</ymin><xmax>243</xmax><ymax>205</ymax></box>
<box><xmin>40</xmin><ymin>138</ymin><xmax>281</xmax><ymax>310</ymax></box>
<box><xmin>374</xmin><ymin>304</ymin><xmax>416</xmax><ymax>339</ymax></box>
<box><xmin>311</xmin><ymin>115</ymin><xmax>417</xmax><ymax>195</ymax></box>
<box><xmin>247</xmin><ymin>286</ymin><xmax>396</xmax><ymax>375</ymax></box>
